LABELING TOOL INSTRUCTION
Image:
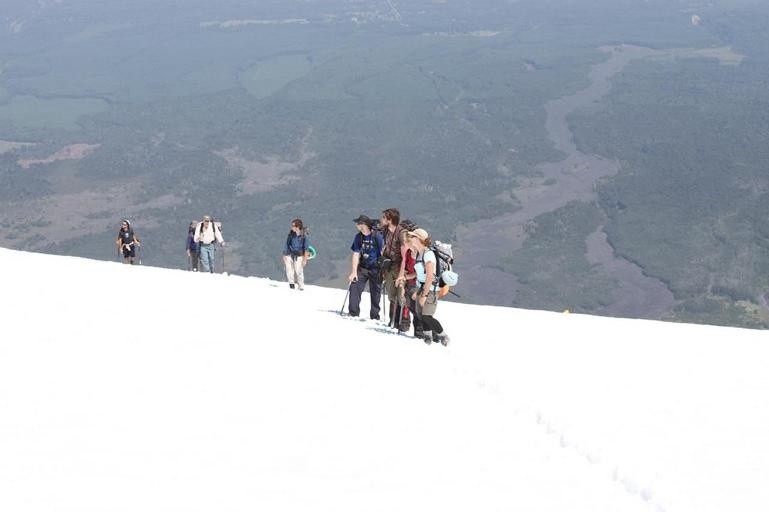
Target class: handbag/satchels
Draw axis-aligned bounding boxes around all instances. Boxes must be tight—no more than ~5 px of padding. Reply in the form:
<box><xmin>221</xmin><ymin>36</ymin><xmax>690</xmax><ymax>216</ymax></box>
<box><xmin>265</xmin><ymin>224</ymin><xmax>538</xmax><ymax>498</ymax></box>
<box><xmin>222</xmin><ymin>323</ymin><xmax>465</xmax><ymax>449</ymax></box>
<box><xmin>377</xmin><ymin>256</ymin><xmax>392</xmax><ymax>270</ymax></box>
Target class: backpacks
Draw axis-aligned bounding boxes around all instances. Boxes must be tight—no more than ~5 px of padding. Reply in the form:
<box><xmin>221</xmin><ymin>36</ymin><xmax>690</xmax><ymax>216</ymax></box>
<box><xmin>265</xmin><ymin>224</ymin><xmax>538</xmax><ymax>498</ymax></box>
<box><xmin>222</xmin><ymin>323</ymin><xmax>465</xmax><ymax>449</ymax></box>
<box><xmin>384</xmin><ymin>218</ymin><xmax>419</xmax><ymax>256</ymax></box>
<box><xmin>287</xmin><ymin>226</ymin><xmax>311</xmax><ymax>252</ymax></box>
<box><xmin>358</xmin><ymin>217</ymin><xmax>382</xmax><ymax>261</ymax></box>
<box><xmin>415</xmin><ymin>241</ymin><xmax>454</xmax><ymax>288</ymax></box>
<box><xmin>200</xmin><ymin>221</ymin><xmax>222</xmax><ymax>244</ymax></box>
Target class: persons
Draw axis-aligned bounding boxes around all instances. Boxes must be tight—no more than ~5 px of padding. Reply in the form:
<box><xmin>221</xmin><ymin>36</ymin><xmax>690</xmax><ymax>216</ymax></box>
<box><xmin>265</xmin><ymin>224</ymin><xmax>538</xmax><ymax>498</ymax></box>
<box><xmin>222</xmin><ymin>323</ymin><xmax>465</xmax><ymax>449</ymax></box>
<box><xmin>117</xmin><ymin>219</ymin><xmax>141</xmax><ymax>265</ymax></box>
<box><xmin>185</xmin><ymin>221</ymin><xmax>201</xmax><ymax>271</ymax></box>
<box><xmin>281</xmin><ymin>219</ymin><xmax>310</xmax><ymax>291</ymax></box>
<box><xmin>346</xmin><ymin>207</ymin><xmax>450</xmax><ymax>346</ymax></box>
<box><xmin>192</xmin><ymin>214</ymin><xmax>227</xmax><ymax>274</ymax></box>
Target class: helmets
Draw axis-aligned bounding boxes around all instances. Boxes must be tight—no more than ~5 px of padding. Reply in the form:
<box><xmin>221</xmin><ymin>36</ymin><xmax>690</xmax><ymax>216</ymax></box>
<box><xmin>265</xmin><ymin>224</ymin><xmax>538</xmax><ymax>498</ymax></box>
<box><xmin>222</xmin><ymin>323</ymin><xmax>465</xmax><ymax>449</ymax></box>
<box><xmin>306</xmin><ymin>247</ymin><xmax>316</xmax><ymax>261</ymax></box>
<box><xmin>442</xmin><ymin>270</ymin><xmax>458</xmax><ymax>286</ymax></box>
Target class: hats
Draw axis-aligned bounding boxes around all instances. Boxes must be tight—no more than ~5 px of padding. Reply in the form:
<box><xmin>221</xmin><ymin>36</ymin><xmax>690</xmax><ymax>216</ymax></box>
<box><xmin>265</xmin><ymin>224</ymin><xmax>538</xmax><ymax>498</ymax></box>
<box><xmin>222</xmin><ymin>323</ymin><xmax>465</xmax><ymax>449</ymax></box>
<box><xmin>353</xmin><ymin>214</ymin><xmax>371</xmax><ymax>226</ymax></box>
<box><xmin>408</xmin><ymin>228</ymin><xmax>428</xmax><ymax>241</ymax></box>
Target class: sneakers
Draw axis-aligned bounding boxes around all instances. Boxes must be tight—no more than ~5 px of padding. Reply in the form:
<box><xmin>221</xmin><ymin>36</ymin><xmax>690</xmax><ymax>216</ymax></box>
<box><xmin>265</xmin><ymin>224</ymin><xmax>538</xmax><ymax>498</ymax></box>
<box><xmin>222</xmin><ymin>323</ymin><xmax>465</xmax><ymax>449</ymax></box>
<box><xmin>347</xmin><ymin>312</ymin><xmax>450</xmax><ymax>348</ymax></box>
